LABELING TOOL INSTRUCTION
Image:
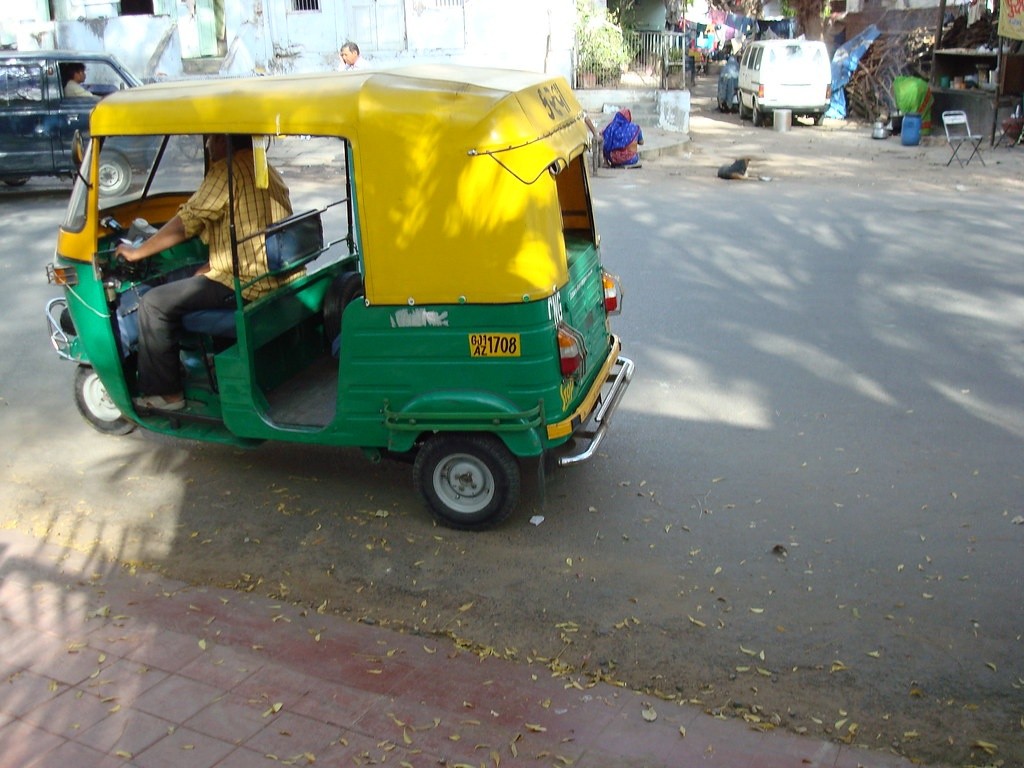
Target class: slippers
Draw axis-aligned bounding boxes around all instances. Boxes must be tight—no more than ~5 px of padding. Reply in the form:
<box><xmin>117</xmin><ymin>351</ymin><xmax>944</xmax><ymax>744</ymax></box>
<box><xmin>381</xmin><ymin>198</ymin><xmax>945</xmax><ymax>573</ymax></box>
<box><xmin>132</xmin><ymin>393</ymin><xmax>187</xmax><ymax>411</ymax></box>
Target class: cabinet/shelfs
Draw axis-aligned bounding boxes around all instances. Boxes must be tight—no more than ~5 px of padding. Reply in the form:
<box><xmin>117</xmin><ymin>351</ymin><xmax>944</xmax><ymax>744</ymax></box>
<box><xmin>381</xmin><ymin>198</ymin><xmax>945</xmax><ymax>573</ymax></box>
<box><xmin>930</xmin><ymin>0</ymin><xmax>1024</xmax><ymax>148</ymax></box>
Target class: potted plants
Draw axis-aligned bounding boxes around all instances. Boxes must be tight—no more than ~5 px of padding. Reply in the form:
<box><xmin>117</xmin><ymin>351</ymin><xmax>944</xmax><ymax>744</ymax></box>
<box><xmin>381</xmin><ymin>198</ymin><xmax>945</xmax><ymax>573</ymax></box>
<box><xmin>661</xmin><ymin>48</ymin><xmax>683</xmax><ymax>89</ymax></box>
<box><xmin>573</xmin><ymin>0</ymin><xmax>643</xmax><ymax>91</ymax></box>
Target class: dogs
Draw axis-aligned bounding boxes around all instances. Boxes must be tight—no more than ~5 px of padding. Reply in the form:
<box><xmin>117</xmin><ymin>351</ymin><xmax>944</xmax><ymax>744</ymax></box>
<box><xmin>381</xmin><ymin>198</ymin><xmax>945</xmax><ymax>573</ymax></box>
<box><xmin>718</xmin><ymin>157</ymin><xmax>759</xmax><ymax>181</ymax></box>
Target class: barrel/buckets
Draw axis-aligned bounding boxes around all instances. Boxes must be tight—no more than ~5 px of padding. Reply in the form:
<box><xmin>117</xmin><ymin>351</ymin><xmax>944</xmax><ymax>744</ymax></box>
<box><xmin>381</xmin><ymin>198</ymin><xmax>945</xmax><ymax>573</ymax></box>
<box><xmin>774</xmin><ymin>110</ymin><xmax>792</xmax><ymax>132</ymax></box>
<box><xmin>901</xmin><ymin>113</ymin><xmax>921</xmax><ymax>145</ymax></box>
<box><xmin>885</xmin><ymin>116</ymin><xmax>903</xmax><ymax>134</ymax></box>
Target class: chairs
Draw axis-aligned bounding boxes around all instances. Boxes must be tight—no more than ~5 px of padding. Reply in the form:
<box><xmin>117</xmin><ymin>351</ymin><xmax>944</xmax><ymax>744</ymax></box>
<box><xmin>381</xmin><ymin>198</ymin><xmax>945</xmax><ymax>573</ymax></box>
<box><xmin>988</xmin><ymin>94</ymin><xmax>1023</xmax><ymax>149</ymax></box>
<box><xmin>942</xmin><ymin>110</ymin><xmax>985</xmax><ymax>169</ymax></box>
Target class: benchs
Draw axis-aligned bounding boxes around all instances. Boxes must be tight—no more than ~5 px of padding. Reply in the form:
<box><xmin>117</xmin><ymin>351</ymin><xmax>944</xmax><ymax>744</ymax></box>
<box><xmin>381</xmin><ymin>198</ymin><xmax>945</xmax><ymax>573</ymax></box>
<box><xmin>181</xmin><ymin>253</ymin><xmax>359</xmax><ymax>360</ymax></box>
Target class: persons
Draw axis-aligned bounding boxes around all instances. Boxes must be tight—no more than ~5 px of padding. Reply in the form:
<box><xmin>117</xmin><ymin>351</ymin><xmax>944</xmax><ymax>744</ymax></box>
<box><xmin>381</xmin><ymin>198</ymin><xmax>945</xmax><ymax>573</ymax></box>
<box><xmin>63</xmin><ymin>63</ymin><xmax>102</xmax><ymax>98</ymax></box>
<box><xmin>340</xmin><ymin>43</ymin><xmax>373</xmax><ymax>70</ymax></box>
<box><xmin>718</xmin><ymin>55</ymin><xmax>739</xmax><ymax>114</ymax></box>
<box><xmin>113</xmin><ymin>133</ymin><xmax>308</xmax><ymax>411</ymax></box>
<box><xmin>598</xmin><ymin>109</ymin><xmax>644</xmax><ymax>168</ymax></box>
